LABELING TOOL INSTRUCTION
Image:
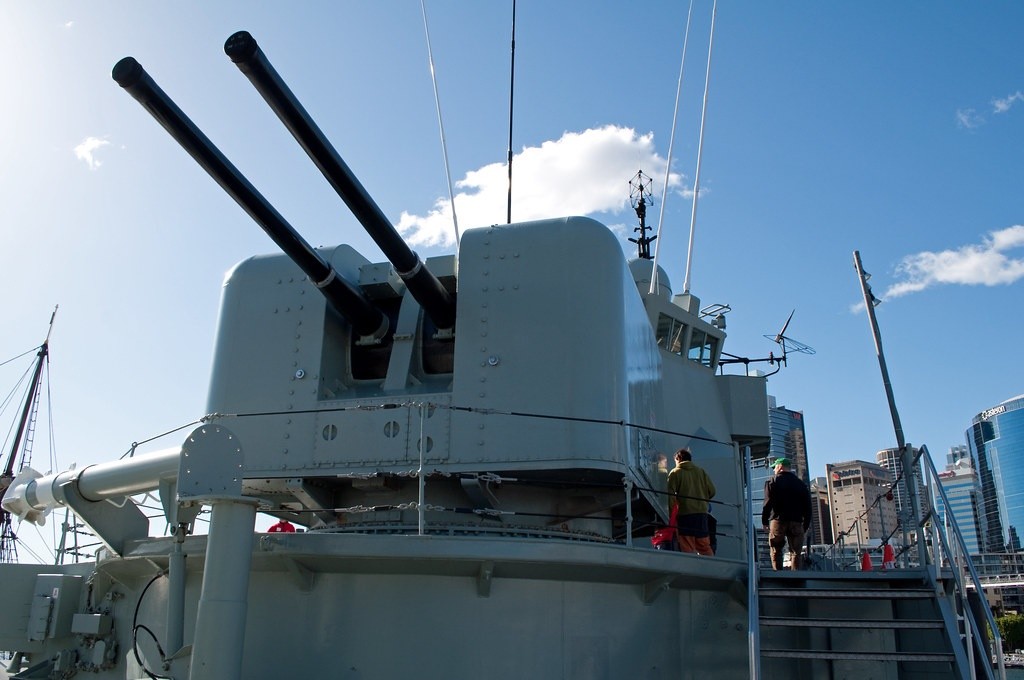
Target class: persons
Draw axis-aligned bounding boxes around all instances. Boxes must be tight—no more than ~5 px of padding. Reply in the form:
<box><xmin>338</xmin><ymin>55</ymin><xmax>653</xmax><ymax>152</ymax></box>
<box><xmin>268</xmin><ymin>519</ymin><xmax>296</xmax><ymax>533</ymax></box>
<box><xmin>763</xmin><ymin>458</ymin><xmax>813</xmax><ymax>570</ymax></box>
<box><xmin>668</xmin><ymin>447</ymin><xmax>716</xmax><ymax>557</ymax></box>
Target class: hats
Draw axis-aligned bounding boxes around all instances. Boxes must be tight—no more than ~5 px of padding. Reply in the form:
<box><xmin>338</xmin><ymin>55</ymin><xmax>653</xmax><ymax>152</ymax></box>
<box><xmin>769</xmin><ymin>457</ymin><xmax>791</xmax><ymax>470</ymax></box>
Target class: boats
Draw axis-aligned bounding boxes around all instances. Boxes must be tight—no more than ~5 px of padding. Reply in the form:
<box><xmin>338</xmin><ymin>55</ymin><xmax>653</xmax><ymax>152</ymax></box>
<box><xmin>990</xmin><ymin>650</ymin><xmax>1024</xmax><ymax>668</ymax></box>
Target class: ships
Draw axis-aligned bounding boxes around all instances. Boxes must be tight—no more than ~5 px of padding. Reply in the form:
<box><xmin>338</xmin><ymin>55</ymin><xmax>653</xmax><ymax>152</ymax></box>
<box><xmin>1</xmin><ymin>0</ymin><xmax>1008</xmax><ymax>680</ymax></box>
